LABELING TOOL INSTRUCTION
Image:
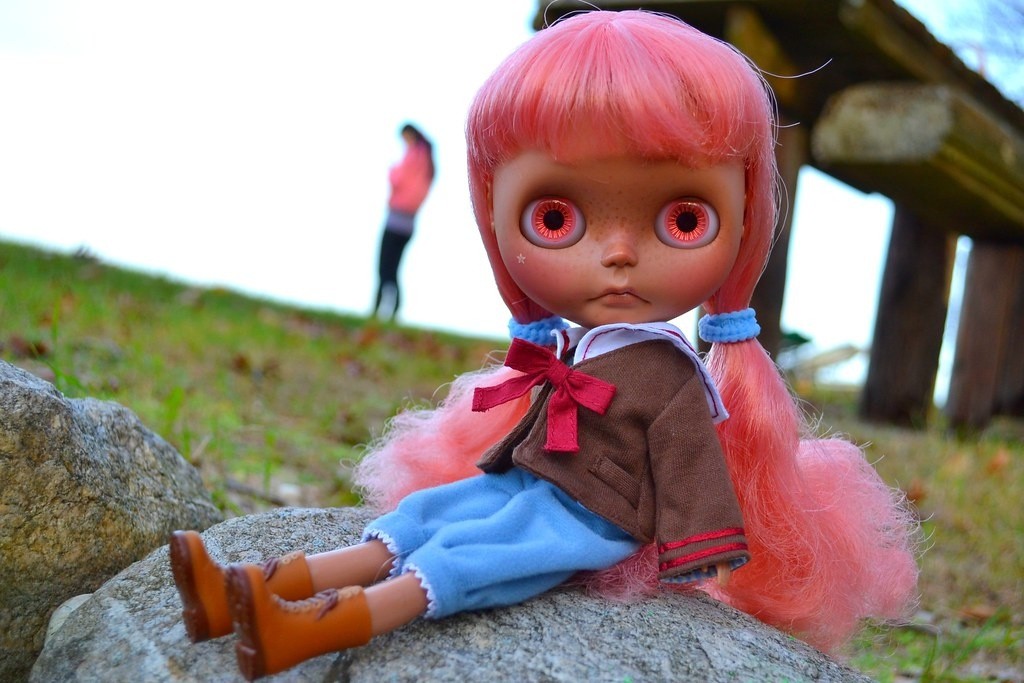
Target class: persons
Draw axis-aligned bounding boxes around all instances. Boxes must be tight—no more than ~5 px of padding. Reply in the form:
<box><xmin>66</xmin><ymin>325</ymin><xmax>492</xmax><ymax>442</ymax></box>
<box><xmin>372</xmin><ymin>125</ymin><xmax>435</xmax><ymax>322</ymax></box>
<box><xmin>169</xmin><ymin>10</ymin><xmax>919</xmax><ymax>683</ymax></box>
